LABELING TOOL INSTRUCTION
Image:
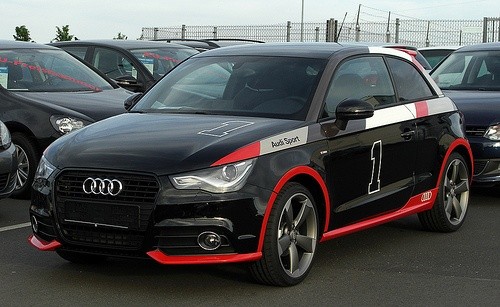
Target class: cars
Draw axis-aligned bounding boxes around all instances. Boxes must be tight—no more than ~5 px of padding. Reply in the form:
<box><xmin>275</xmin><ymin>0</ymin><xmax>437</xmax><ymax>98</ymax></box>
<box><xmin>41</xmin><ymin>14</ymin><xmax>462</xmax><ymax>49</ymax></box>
<box><xmin>28</xmin><ymin>43</ymin><xmax>474</xmax><ymax>287</ymax></box>
<box><xmin>0</xmin><ymin>120</ymin><xmax>19</xmax><ymax>199</ymax></box>
<box><xmin>427</xmin><ymin>42</ymin><xmax>500</xmax><ymax>182</ymax></box>
<box><xmin>47</xmin><ymin>40</ymin><xmax>199</xmax><ymax>95</ymax></box>
<box><xmin>0</xmin><ymin>43</ymin><xmax>144</xmax><ymax>197</ymax></box>
<box><xmin>152</xmin><ymin>39</ymin><xmax>464</xmax><ymax>81</ymax></box>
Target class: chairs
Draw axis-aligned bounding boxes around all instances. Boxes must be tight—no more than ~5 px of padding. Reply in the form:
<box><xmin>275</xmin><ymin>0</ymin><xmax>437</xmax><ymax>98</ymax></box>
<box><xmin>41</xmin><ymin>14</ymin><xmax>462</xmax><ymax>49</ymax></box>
<box><xmin>222</xmin><ymin>68</ymin><xmax>370</xmax><ymax>112</ymax></box>
<box><xmin>7</xmin><ymin>65</ymin><xmax>28</xmax><ymax>88</ymax></box>
